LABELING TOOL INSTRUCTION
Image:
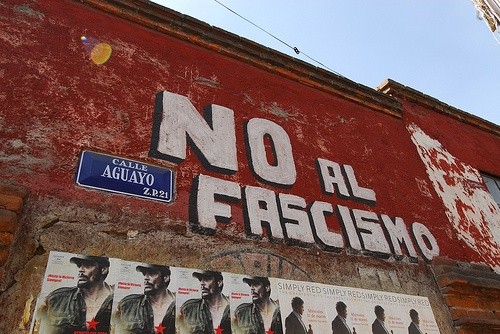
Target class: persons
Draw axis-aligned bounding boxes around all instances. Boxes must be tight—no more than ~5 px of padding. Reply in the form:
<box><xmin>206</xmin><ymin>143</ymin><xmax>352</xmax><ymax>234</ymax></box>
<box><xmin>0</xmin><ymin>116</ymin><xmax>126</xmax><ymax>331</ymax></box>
<box><xmin>231</xmin><ymin>277</ymin><xmax>283</xmax><ymax>334</ymax></box>
<box><xmin>285</xmin><ymin>296</ymin><xmax>313</xmax><ymax>334</ymax></box>
<box><xmin>35</xmin><ymin>255</ymin><xmax>115</xmax><ymax>334</ymax></box>
<box><xmin>332</xmin><ymin>301</ymin><xmax>356</xmax><ymax>334</ymax></box>
<box><xmin>372</xmin><ymin>305</ymin><xmax>389</xmax><ymax>334</ymax></box>
<box><xmin>112</xmin><ymin>264</ymin><xmax>176</xmax><ymax>334</ymax></box>
<box><xmin>175</xmin><ymin>270</ymin><xmax>231</xmax><ymax>334</ymax></box>
<box><xmin>408</xmin><ymin>309</ymin><xmax>424</xmax><ymax>334</ymax></box>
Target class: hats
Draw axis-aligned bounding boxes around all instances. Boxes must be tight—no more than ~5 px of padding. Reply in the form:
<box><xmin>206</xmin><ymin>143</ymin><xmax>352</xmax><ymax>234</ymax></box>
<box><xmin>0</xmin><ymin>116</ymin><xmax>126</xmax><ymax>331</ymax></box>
<box><xmin>192</xmin><ymin>270</ymin><xmax>223</xmax><ymax>281</ymax></box>
<box><xmin>136</xmin><ymin>264</ymin><xmax>171</xmax><ymax>275</ymax></box>
<box><xmin>243</xmin><ymin>276</ymin><xmax>270</xmax><ymax>285</ymax></box>
<box><xmin>70</xmin><ymin>254</ymin><xmax>110</xmax><ymax>268</ymax></box>
